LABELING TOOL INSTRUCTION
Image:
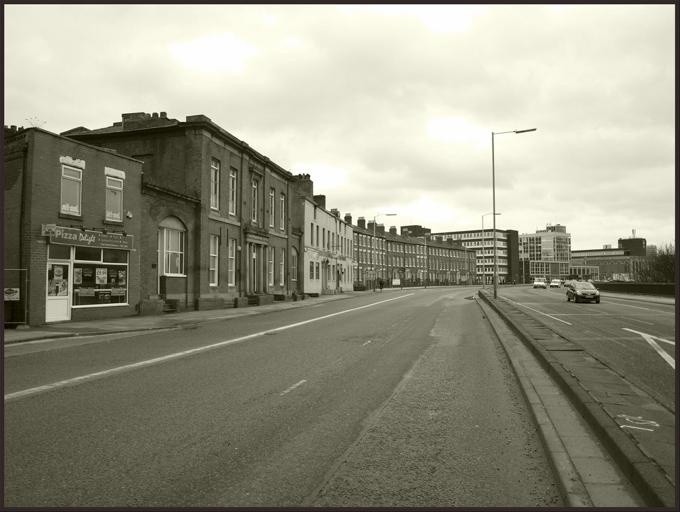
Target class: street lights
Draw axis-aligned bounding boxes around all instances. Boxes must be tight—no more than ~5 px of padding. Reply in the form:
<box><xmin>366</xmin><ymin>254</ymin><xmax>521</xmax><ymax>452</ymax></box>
<box><xmin>372</xmin><ymin>213</ymin><xmax>398</xmax><ymax>292</ymax></box>
<box><xmin>492</xmin><ymin>126</ymin><xmax>537</xmax><ymax>301</ymax></box>
<box><xmin>482</xmin><ymin>212</ymin><xmax>501</xmax><ymax>289</ymax></box>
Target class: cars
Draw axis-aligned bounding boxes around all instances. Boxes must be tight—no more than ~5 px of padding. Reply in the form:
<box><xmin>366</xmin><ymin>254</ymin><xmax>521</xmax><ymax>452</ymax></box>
<box><xmin>533</xmin><ymin>278</ymin><xmax>624</xmax><ymax>288</ymax></box>
<box><xmin>566</xmin><ymin>281</ymin><xmax>600</xmax><ymax>303</ymax></box>
